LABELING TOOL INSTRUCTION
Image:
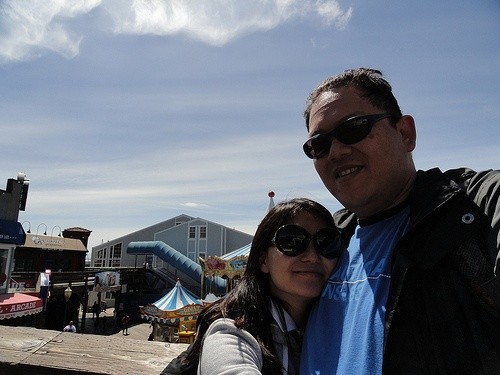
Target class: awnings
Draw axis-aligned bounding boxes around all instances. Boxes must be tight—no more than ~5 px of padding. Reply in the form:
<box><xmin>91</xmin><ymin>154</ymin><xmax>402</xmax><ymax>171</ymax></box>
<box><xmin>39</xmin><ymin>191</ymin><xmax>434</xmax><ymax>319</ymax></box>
<box><xmin>0</xmin><ymin>293</ymin><xmax>43</xmax><ymax>320</ymax></box>
<box><xmin>0</xmin><ymin>219</ymin><xmax>26</xmax><ymax>245</ymax></box>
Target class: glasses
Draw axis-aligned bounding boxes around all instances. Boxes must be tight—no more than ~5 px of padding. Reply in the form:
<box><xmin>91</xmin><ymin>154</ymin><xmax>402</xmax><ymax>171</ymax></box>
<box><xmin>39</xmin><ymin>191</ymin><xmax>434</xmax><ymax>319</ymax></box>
<box><xmin>265</xmin><ymin>225</ymin><xmax>343</xmax><ymax>259</ymax></box>
<box><xmin>303</xmin><ymin>113</ymin><xmax>400</xmax><ymax>159</ymax></box>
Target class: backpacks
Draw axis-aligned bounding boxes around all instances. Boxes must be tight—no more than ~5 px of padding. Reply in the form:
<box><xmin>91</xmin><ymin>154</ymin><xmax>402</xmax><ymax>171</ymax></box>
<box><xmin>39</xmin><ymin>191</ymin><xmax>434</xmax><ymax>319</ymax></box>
<box><xmin>160</xmin><ymin>306</ymin><xmax>283</xmax><ymax>375</ymax></box>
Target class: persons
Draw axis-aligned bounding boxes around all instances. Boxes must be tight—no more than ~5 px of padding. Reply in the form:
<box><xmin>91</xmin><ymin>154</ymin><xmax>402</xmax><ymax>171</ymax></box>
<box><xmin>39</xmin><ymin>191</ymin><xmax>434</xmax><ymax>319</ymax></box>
<box><xmin>91</xmin><ymin>301</ymin><xmax>100</xmax><ymax>318</ymax></box>
<box><xmin>114</xmin><ymin>312</ymin><xmax>129</xmax><ymax>335</ymax></box>
<box><xmin>297</xmin><ymin>68</ymin><xmax>500</xmax><ymax>375</ymax></box>
<box><xmin>194</xmin><ymin>197</ymin><xmax>345</xmax><ymax>375</ymax></box>
<box><xmin>102</xmin><ymin>316</ymin><xmax>107</xmax><ymax>332</ymax></box>
<box><xmin>63</xmin><ymin>321</ymin><xmax>76</xmax><ymax>333</ymax></box>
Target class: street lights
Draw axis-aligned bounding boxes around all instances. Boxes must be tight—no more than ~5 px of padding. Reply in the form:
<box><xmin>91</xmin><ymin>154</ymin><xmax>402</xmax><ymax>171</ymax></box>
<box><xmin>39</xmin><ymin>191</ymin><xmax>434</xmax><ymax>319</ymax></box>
<box><xmin>21</xmin><ymin>220</ymin><xmax>32</xmax><ymax>233</ymax></box>
<box><xmin>51</xmin><ymin>225</ymin><xmax>62</xmax><ymax>237</ymax></box>
<box><xmin>36</xmin><ymin>223</ymin><xmax>48</xmax><ymax>235</ymax></box>
